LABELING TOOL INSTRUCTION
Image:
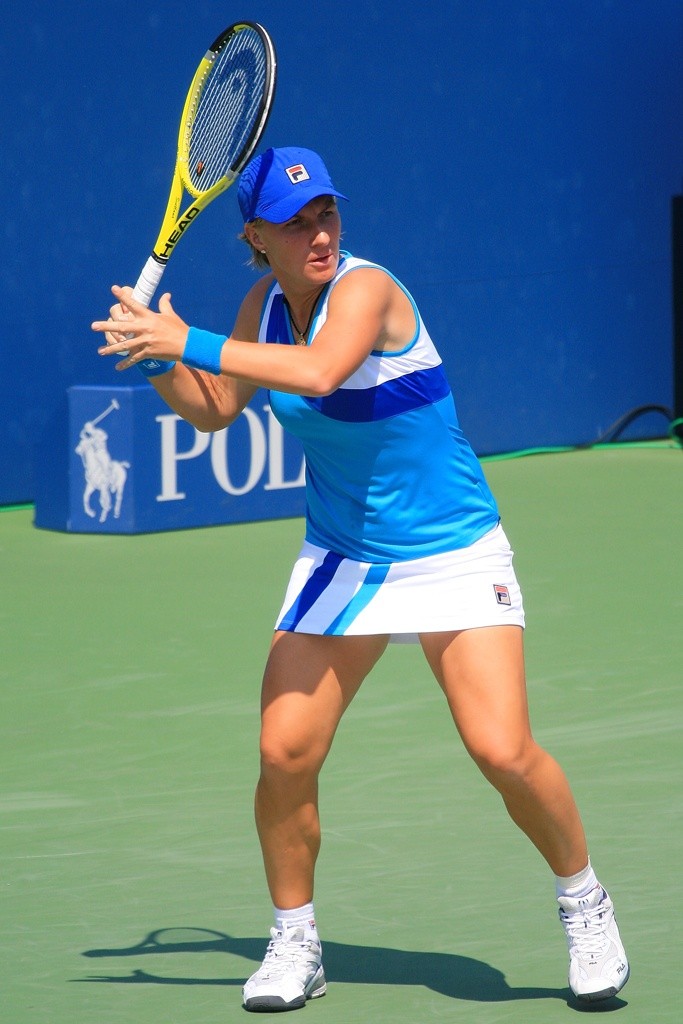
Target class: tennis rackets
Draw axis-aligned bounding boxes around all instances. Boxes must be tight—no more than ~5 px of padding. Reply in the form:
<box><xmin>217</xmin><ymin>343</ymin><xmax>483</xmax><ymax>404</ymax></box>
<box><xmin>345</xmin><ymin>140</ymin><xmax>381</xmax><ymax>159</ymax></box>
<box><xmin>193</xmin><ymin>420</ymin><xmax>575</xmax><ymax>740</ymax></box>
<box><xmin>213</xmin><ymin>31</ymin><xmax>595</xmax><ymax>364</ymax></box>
<box><xmin>113</xmin><ymin>19</ymin><xmax>279</xmax><ymax>356</ymax></box>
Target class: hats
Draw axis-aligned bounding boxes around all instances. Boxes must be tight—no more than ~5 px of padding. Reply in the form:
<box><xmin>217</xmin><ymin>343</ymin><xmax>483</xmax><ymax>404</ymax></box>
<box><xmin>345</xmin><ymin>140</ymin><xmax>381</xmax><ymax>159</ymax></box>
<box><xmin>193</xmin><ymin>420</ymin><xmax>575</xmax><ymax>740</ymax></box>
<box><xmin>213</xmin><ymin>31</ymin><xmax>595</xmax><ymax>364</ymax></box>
<box><xmin>238</xmin><ymin>147</ymin><xmax>350</xmax><ymax>225</ymax></box>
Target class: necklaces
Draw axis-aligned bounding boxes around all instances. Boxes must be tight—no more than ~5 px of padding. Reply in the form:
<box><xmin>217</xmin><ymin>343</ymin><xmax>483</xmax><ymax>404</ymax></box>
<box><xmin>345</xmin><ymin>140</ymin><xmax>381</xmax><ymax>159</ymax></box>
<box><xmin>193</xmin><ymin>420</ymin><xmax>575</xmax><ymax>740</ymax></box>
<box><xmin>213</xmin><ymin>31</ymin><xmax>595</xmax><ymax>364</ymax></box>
<box><xmin>285</xmin><ymin>285</ymin><xmax>325</xmax><ymax>345</ymax></box>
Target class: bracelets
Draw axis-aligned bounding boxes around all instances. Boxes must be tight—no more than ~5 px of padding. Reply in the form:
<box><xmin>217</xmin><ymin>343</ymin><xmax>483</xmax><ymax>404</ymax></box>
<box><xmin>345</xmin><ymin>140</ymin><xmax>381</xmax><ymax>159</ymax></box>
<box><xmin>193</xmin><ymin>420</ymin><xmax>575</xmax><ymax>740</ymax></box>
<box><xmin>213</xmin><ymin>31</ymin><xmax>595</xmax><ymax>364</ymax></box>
<box><xmin>183</xmin><ymin>327</ymin><xmax>228</xmax><ymax>376</ymax></box>
<box><xmin>135</xmin><ymin>359</ymin><xmax>176</xmax><ymax>377</ymax></box>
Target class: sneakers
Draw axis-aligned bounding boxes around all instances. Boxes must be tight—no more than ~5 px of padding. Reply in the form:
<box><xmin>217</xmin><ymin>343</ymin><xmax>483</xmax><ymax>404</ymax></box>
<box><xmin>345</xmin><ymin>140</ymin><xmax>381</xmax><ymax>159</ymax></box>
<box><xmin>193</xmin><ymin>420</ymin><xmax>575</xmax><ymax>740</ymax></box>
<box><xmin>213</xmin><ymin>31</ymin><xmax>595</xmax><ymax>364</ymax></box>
<box><xmin>556</xmin><ymin>883</ymin><xmax>630</xmax><ymax>1001</ymax></box>
<box><xmin>241</xmin><ymin>921</ymin><xmax>327</xmax><ymax>1011</ymax></box>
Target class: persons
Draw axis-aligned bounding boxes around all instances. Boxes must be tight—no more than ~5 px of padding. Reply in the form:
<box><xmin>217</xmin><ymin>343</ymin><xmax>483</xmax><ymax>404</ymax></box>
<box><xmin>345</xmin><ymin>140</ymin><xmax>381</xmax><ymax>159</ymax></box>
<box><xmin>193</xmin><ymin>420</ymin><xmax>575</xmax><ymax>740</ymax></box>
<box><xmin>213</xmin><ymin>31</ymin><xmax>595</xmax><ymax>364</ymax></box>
<box><xmin>90</xmin><ymin>146</ymin><xmax>631</xmax><ymax>1011</ymax></box>
